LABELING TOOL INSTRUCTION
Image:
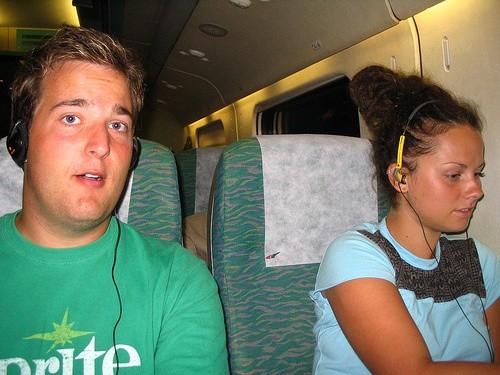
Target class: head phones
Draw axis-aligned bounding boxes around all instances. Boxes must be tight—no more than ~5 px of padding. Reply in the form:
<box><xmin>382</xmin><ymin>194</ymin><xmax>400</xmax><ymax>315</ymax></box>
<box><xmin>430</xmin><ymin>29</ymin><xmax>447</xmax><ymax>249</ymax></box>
<box><xmin>3</xmin><ymin>50</ymin><xmax>142</xmax><ymax>169</ymax></box>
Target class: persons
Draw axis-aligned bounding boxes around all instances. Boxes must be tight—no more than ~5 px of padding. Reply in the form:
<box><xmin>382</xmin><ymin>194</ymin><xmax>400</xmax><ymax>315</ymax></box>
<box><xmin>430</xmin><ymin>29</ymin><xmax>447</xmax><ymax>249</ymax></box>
<box><xmin>308</xmin><ymin>65</ymin><xmax>500</xmax><ymax>375</ymax></box>
<box><xmin>184</xmin><ymin>209</ymin><xmax>206</xmax><ymax>266</ymax></box>
<box><xmin>3</xmin><ymin>21</ymin><xmax>230</xmax><ymax>375</ymax></box>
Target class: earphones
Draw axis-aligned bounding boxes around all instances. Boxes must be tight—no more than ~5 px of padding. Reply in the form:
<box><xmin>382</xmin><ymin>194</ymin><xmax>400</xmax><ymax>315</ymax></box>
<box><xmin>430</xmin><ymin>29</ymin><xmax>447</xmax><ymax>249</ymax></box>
<box><xmin>394</xmin><ymin>98</ymin><xmax>444</xmax><ymax>184</ymax></box>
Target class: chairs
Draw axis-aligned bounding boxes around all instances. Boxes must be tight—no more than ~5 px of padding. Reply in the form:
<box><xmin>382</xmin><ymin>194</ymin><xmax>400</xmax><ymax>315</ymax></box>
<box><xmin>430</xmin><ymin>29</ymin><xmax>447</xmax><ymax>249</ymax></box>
<box><xmin>0</xmin><ymin>133</ymin><xmax>390</xmax><ymax>375</ymax></box>
